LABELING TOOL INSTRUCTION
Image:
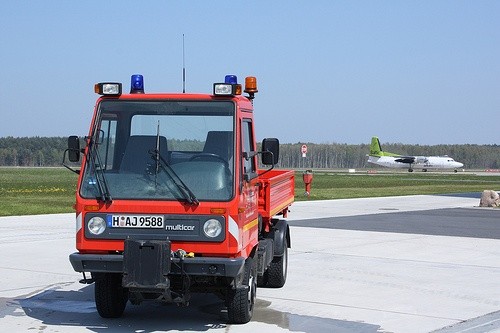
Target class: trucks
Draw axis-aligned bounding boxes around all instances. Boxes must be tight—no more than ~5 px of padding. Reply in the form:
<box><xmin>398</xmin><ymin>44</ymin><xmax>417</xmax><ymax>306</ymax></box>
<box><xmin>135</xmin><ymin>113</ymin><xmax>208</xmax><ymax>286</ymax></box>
<box><xmin>61</xmin><ymin>73</ymin><xmax>296</xmax><ymax>324</ymax></box>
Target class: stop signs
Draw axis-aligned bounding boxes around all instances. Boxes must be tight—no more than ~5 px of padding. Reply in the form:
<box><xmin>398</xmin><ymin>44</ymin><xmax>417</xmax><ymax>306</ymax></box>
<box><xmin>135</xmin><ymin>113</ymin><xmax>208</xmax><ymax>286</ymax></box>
<box><xmin>300</xmin><ymin>144</ymin><xmax>308</xmax><ymax>154</ymax></box>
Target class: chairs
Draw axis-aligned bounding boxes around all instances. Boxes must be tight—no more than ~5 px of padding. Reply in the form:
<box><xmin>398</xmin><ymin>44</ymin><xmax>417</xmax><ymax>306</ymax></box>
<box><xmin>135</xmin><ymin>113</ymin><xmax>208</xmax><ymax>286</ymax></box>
<box><xmin>119</xmin><ymin>135</ymin><xmax>168</xmax><ymax>174</ymax></box>
<box><xmin>202</xmin><ymin>130</ymin><xmax>234</xmax><ymax>161</ymax></box>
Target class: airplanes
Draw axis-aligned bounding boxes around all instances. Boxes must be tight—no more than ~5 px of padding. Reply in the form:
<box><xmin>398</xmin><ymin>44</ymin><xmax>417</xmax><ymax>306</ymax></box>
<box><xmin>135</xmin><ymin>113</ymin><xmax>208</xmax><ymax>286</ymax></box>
<box><xmin>364</xmin><ymin>136</ymin><xmax>464</xmax><ymax>172</ymax></box>
